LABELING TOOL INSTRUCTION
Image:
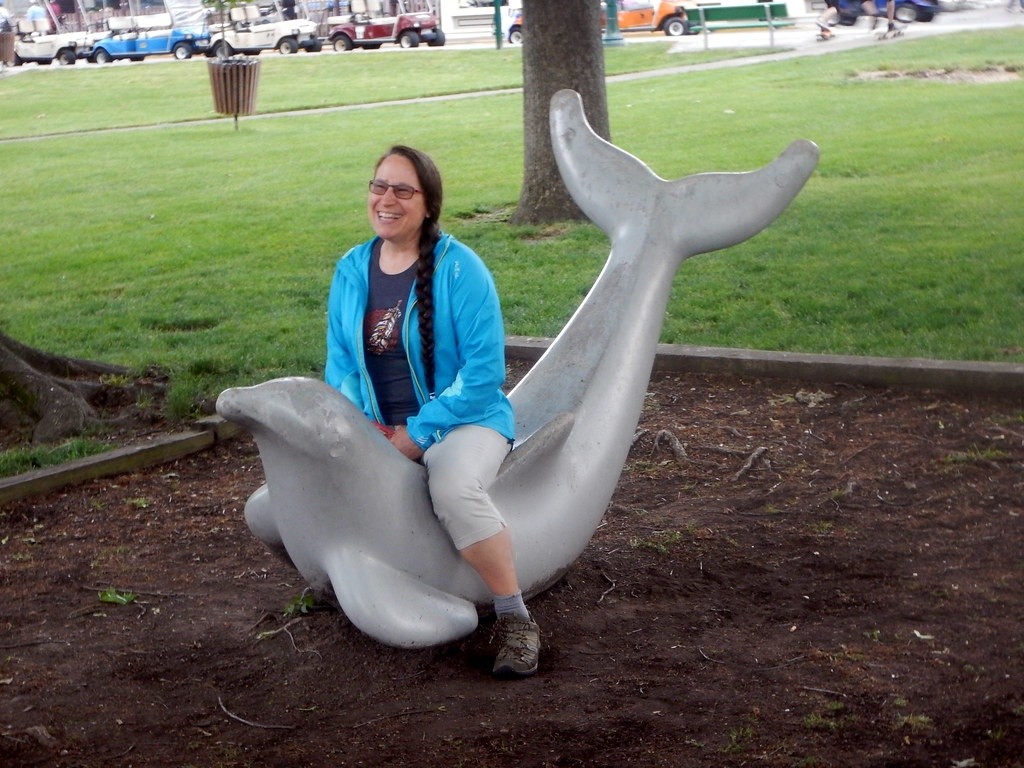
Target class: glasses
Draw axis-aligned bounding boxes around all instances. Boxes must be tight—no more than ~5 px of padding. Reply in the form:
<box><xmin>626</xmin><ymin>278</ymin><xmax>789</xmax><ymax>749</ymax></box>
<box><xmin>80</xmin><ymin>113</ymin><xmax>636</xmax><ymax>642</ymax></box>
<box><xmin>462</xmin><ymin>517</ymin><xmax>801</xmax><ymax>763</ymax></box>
<box><xmin>369</xmin><ymin>180</ymin><xmax>424</xmax><ymax>199</ymax></box>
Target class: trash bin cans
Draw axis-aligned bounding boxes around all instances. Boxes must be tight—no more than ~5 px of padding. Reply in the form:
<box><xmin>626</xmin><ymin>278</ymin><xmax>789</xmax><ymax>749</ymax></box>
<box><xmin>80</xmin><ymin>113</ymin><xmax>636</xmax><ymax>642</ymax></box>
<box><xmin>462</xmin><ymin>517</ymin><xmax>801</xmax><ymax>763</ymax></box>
<box><xmin>207</xmin><ymin>57</ymin><xmax>262</xmax><ymax>116</ymax></box>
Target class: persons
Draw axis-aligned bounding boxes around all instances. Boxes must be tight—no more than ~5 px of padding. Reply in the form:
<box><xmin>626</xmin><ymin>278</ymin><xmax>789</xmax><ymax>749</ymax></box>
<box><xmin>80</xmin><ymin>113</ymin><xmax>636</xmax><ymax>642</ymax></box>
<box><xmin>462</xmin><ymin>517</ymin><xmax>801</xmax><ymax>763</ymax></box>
<box><xmin>0</xmin><ymin>0</ymin><xmax>128</xmax><ymax>67</ymax></box>
<box><xmin>815</xmin><ymin>0</ymin><xmax>1024</xmax><ymax>40</ymax></box>
<box><xmin>281</xmin><ymin>0</ymin><xmax>298</xmax><ymax>20</ymax></box>
<box><xmin>326</xmin><ymin>145</ymin><xmax>551</xmax><ymax>679</ymax></box>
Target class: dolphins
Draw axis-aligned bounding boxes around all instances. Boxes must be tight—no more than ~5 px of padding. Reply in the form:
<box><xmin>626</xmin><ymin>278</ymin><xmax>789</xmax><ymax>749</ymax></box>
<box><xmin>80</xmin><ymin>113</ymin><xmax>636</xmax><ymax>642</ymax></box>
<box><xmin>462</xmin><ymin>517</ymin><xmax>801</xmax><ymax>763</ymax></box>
<box><xmin>215</xmin><ymin>91</ymin><xmax>820</xmax><ymax>647</ymax></box>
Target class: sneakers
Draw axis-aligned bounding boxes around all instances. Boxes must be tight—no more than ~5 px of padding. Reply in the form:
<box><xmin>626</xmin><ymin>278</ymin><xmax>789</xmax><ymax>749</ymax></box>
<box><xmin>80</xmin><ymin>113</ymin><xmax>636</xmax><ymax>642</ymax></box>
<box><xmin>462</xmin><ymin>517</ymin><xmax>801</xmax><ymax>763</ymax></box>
<box><xmin>302</xmin><ymin>585</ymin><xmax>331</xmax><ymax>607</ymax></box>
<box><xmin>487</xmin><ymin>610</ymin><xmax>542</xmax><ymax>676</ymax></box>
